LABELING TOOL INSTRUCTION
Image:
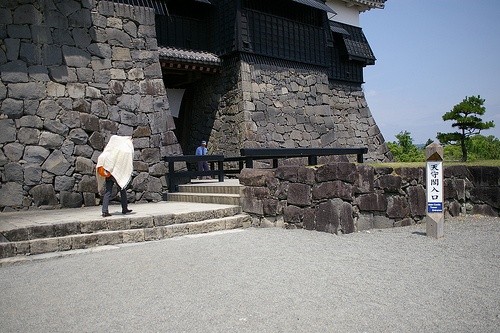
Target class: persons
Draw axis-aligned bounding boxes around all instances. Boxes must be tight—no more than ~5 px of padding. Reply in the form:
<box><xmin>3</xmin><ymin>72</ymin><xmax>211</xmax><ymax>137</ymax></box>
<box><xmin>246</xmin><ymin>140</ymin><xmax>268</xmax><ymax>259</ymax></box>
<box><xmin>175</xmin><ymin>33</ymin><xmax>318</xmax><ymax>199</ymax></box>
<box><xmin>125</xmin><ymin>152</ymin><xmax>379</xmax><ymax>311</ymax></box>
<box><xmin>195</xmin><ymin>139</ymin><xmax>211</xmax><ymax>180</ymax></box>
<box><xmin>95</xmin><ymin>135</ymin><xmax>134</xmax><ymax>217</ymax></box>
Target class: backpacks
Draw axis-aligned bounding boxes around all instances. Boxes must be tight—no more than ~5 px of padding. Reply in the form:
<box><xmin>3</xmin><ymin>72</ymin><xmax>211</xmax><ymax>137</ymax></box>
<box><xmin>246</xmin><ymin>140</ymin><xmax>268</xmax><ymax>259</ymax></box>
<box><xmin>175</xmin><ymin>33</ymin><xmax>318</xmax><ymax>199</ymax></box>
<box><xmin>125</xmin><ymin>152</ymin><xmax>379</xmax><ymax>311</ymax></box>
<box><xmin>98</xmin><ymin>167</ymin><xmax>111</xmax><ymax>177</ymax></box>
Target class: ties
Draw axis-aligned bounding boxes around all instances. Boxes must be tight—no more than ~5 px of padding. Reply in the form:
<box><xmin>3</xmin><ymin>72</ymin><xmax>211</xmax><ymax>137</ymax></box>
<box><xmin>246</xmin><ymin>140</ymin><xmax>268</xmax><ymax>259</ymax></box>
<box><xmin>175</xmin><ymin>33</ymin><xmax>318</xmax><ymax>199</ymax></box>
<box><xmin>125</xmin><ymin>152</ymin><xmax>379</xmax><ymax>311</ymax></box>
<box><xmin>202</xmin><ymin>148</ymin><xmax>205</xmax><ymax>155</ymax></box>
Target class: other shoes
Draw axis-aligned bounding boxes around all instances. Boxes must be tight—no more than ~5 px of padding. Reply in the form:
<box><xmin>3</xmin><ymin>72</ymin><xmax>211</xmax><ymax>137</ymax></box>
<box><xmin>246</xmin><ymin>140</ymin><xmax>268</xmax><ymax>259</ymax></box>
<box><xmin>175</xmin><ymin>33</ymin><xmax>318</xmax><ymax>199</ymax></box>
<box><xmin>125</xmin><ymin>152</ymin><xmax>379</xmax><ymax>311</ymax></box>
<box><xmin>103</xmin><ymin>213</ymin><xmax>112</xmax><ymax>217</ymax></box>
<box><xmin>122</xmin><ymin>209</ymin><xmax>132</xmax><ymax>214</ymax></box>
